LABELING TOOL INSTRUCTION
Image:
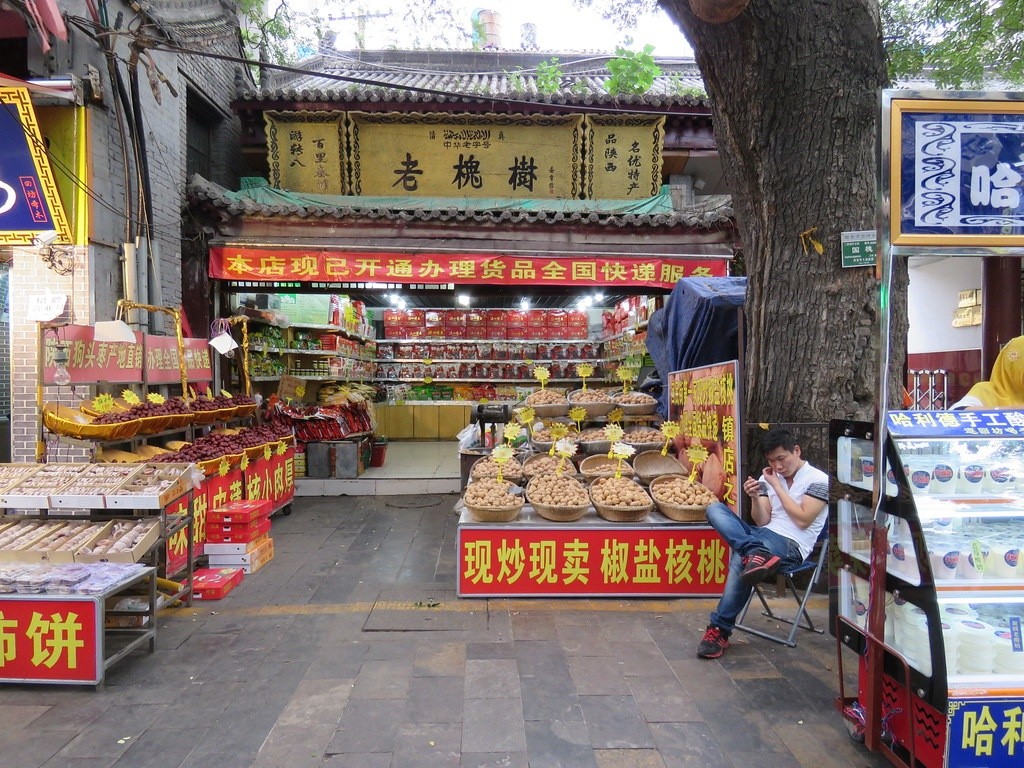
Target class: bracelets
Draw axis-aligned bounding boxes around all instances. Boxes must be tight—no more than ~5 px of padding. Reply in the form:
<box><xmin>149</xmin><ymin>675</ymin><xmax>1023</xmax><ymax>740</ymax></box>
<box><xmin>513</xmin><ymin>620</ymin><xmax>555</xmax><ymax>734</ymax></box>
<box><xmin>996</xmin><ymin>407</ymin><xmax>999</xmax><ymax>410</ymax></box>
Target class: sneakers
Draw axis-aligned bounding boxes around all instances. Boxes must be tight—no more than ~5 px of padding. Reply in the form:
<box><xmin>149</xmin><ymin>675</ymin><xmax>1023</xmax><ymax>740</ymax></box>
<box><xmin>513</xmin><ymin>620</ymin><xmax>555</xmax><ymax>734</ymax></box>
<box><xmin>738</xmin><ymin>547</ymin><xmax>781</xmax><ymax>586</ymax></box>
<box><xmin>697</xmin><ymin>625</ymin><xmax>731</xmax><ymax>658</ymax></box>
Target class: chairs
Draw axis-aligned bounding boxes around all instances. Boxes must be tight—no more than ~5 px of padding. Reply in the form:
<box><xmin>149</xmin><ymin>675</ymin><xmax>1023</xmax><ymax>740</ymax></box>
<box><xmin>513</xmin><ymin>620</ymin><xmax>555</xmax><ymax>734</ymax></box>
<box><xmin>733</xmin><ymin>517</ymin><xmax>829</xmax><ymax>648</ymax></box>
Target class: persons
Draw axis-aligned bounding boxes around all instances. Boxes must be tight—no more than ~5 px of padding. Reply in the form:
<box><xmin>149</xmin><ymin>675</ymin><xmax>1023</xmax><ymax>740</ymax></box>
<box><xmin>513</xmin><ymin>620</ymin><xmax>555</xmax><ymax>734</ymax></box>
<box><xmin>948</xmin><ymin>335</ymin><xmax>1024</xmax><ymax>410</ymax></box>
<box><xmin>696</xmin><ymin>429</ymin><xmax>829</xmax><ymax>658</ymax></box>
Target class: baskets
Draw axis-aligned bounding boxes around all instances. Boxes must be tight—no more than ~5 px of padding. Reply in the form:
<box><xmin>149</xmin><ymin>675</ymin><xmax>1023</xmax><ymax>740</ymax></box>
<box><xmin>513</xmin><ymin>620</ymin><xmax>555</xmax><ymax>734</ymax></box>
<box><xmin>579</xmin><ymin>454</ymin><xmax>634</xmax><ymax>485</ymax></box>
<box><xmin>632</xmin><ymin>450</ymin><xmax>689</xmax><ymax>485</ymax></box>
<box><xmin>525</xmin><ymin>473</ymin><xmax>589</xmax><ymax>521</ymax></box>
<box><xmin>463</xmin><ymin>478</ymin><xmax>525</xmax><ymax>521</ymax></box>
<box><xmin>567</xmin><ymin>388</ymin><xmax>616</xmax><ymax>416</ymax></box>
<box><xmin>522</xmin><ymin>452</ymin><xmax>577</xmax><ymax>483</ymax></box>
<box><xmin>580</xmin><ymin>428</ymin><xmax>619</xmax><ymax>455</ymax></box>
<box><xmin>42</xmin><ymin>396</ymin><xmax>257</xmax><ymax>439</ymax></box>
<box><xmin>531</xmin><ymin>422</ymin><xmax>581</xmax><ymax>452</ymax></box>
<box><xmin>612</xmin><ymin>391</ymin><xmax>658</xmax><ymax>415</ymax></box>
<box><xmin>589</xmin><ymin>474</ymin><xmax>653</xmax><ymax>522</ymax></box>
<box><xmin>620</xmin><ymin>425</ymin><xmax>673</xmax><ymax>454</ymax></box>
<box><xmin>525</xmin><ymin>391</ymin><xmax>569</xmax><ymax>417</ymax></box>
<box><xmin>470</xmin><ymin>453</ymin><xmax>523</xmax><ymax>485</ymax></box>
<box><xmin>97</xmin><ymin>427</ymin><xmax>295</xmax><ymax>476</ymax></box>
<box><xmin>649</xmin><ymin>473</ymin><xmax>719</xmax><ymax>521</ymax></box>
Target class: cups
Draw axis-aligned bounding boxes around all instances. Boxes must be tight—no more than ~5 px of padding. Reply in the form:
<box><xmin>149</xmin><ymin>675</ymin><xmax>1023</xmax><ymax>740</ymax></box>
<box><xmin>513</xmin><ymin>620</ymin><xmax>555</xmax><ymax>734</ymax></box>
<box><xmin>858</xmin><ymin>453</ymin><xmax>1024</xmax><ymax>677</ymax></box>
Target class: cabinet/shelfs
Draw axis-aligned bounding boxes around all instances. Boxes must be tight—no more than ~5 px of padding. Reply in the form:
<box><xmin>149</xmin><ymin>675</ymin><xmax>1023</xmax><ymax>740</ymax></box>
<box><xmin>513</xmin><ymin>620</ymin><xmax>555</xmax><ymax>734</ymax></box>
<box><xmin>370</xmin><ymin>320</ymin><xmax>655</xmax><ymax>444</ymax></box>
<box><xmin>846</xmin><ymin>479</ymin><xmax>1024</xmax><ymax>676</ymax></box>
<box><xmin>0</xmin><ymin>462</ymin><xmax>196</xmax><ymax>692</ymax></box>
<box><xmin>36</xmin><ymin>319</ymin><xmax>296</xmax><ymax>579</ymax></box>
<box><xmin>232</xmin><ymin>317</ymin><xmax>377</xmax><ymax>384</ymax></box>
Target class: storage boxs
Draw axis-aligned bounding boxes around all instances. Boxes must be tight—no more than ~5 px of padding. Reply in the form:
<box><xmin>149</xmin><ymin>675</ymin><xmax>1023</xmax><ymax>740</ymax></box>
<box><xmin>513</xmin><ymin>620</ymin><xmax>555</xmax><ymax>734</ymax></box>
<box><xmin>323</xmin><ymin>296</ymin><xmax>376</xmax><ymax>379</ymax></box>
<box><xmin>294</xmin><ymin>454</ymin><xmax>308</xmax><ymax>477</ymax></box>
<box><xmin>174</xmin><ymin>499</ymin><xmax>274</xmax><ymax>601</ymax></box>
<box><xmin>104</xmin><ymin>595</ymin><xmax>163</xmax><ymax>626</ymax></box>
<box><xmin>384</xmin><ymin>294</ymin><xmax>662</xmax><ymax>340</ymax></box>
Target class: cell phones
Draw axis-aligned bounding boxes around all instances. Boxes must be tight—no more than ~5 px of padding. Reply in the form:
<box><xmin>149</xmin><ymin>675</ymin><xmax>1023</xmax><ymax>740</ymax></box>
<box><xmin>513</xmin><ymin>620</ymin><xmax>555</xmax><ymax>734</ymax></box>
<box><xmin>755</xmin><ymin>481</ymin><xmax>770</xmax><ymax>491</ymax></box>
